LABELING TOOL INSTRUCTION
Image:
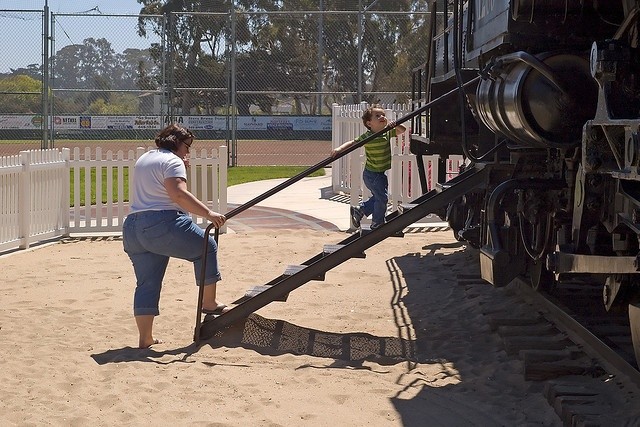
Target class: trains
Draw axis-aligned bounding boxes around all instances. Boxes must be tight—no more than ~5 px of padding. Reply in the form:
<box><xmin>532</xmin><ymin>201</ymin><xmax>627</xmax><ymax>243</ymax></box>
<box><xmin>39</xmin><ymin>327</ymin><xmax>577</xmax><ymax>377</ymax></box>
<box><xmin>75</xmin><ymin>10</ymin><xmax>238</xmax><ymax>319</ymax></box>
<box><xmin>408</xmin><ymin>0</ymin><xmax>635</xmax><ymax>384</ymax></box>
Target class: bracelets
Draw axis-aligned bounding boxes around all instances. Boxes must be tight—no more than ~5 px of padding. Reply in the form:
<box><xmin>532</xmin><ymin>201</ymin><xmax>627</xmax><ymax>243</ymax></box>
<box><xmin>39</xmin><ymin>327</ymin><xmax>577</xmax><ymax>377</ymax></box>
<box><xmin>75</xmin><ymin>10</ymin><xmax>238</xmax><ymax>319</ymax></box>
<box><xmin>205</xmin><ymin>210</ymin><xmax>212</xmax><ymax>219</ymax></box>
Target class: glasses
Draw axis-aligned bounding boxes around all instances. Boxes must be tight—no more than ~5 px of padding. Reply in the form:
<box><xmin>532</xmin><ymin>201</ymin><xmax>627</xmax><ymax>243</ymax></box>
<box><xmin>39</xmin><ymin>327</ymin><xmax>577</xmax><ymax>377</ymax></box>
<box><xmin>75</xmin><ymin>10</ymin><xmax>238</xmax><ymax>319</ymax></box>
<box><xmin>182</xmin><ymin>140</ymin><xmax>190</xmax><ymax>147</ymax></box>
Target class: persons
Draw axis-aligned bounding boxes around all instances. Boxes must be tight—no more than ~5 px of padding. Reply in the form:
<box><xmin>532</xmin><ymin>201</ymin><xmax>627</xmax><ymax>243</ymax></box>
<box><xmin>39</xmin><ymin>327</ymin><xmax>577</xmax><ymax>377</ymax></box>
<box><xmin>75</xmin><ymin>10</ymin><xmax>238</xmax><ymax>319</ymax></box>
<box><xmin>122</xmin><ymin>125</ymin><xmax>233</xmax><ymax>348</ymax></box>
<box><xmin>330</xmin><ymin>107</ymin><xmax>406</xmax><ymax>231</ymax></box>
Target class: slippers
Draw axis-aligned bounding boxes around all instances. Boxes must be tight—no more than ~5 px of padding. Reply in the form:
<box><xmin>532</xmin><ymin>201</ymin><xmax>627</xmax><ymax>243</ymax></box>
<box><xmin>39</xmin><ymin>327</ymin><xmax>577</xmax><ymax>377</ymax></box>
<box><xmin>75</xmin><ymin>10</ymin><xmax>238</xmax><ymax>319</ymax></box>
<box><xmin>202</xmin><ymin>305</ymin><xmax>227</xmax><ymax>315</ymax></box>
<box><xmin>146</xmin><ymin>339</ymin><xmax>158</xmax><ymax>348</ymax></box>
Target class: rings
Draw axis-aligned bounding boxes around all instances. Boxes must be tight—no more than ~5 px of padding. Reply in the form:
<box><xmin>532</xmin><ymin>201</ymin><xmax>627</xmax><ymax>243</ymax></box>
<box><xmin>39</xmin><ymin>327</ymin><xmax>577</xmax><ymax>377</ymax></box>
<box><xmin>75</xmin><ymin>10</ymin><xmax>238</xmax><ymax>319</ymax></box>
<box><xmin>220</xmin><ymin>217</ymin><xmax>221</xmax><ymax>219</ymax></box>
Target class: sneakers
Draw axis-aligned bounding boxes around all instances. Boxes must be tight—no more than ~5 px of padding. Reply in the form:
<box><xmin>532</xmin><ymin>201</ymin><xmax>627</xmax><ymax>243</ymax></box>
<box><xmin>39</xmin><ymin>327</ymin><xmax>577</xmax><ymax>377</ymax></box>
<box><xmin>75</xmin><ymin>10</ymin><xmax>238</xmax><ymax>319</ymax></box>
<box><xmin>370</xmin><ymin>222</ymin><xmax>381</xmax><ymax>231</ymax></box>
<box><xmin>351</xmin><ymin>205</ymin><xmax>364</xmax><ymax>228</ymax></box>
